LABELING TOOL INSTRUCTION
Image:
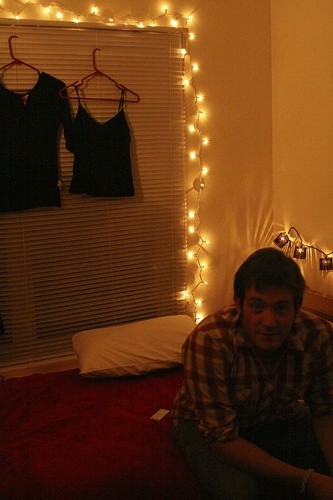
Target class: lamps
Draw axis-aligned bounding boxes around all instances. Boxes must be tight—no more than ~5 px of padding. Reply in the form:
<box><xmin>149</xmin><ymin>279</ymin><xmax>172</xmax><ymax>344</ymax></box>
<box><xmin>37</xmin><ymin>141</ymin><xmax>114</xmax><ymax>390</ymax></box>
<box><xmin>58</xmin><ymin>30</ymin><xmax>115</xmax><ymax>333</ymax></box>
<box><xmin>274</xmin><ymin>226</ymin><xmax>333</xmax><ymax>271</ymax></box>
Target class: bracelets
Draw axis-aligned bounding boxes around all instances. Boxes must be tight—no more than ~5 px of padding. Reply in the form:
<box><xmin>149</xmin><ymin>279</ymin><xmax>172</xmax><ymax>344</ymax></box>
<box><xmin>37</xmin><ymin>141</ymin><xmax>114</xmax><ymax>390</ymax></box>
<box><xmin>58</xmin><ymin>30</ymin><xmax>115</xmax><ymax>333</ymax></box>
<box><xmin>300</xmin><ymin>468</ymin><xmax>315</xmax><ymax>493</ymax></box>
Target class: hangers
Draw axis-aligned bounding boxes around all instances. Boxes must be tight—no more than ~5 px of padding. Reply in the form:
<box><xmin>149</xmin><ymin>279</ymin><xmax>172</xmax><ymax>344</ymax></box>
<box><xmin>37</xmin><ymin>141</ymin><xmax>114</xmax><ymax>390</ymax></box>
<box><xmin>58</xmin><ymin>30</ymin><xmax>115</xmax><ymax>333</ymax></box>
<box><xmin>58</xmin><ymin>48</ymin><xmax>141</xmax><ymax>103</ymax></box>
<box><xmin>0</xmin><ymin>35</ymin><xmax>41</xmax><ymax>96</ymax></box>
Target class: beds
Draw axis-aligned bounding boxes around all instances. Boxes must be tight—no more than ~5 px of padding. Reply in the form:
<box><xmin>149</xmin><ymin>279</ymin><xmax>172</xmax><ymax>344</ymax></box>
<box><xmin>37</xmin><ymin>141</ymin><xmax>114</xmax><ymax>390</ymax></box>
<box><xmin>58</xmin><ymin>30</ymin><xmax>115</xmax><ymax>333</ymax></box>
<box><xmin>0</xmin><ymin>366</ymin><xmax>207</xmax><ymax>500</ymax></box>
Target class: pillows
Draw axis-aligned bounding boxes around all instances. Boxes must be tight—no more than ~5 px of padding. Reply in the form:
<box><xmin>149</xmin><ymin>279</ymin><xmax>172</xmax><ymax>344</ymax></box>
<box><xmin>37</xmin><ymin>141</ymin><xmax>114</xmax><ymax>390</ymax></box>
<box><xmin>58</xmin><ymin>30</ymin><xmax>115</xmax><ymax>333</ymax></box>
<box><xmin>71</xmin><ymin>314</ymin><xmax>198</xmax><ymax>380</ymax></box>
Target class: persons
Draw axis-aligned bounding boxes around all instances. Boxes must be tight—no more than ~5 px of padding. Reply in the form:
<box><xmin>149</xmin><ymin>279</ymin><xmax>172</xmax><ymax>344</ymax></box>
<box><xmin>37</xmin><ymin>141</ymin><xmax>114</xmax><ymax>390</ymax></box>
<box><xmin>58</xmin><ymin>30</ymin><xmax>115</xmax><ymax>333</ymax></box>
<box><xmin>170</xmin><ymin>247</ymin><xmax>333</xmax><ymax>500</ymax></box>
